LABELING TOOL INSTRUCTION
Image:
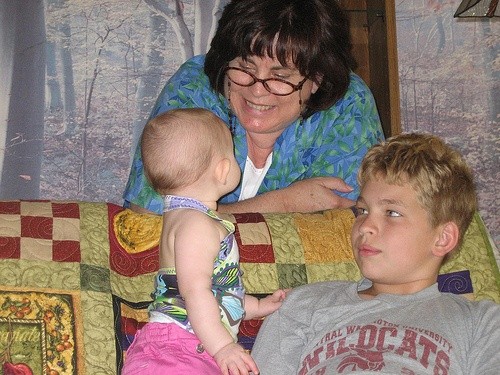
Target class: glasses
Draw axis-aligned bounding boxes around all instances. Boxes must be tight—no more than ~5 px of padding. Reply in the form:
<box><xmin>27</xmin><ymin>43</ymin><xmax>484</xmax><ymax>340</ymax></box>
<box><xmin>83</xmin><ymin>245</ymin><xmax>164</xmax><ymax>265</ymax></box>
<box><xmin>224</xmin><ymin>62</ymin><xmax>310</xmax><ymax>96</ymax></box>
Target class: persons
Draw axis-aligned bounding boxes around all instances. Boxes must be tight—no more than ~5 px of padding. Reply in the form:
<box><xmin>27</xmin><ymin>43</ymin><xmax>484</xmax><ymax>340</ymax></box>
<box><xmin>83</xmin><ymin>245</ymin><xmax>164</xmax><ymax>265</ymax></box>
<box><xmin>250</xmin><ymin>131</ymin><xmax>500</xmax><ymax>375</ymax></box>
<box><xmin>120</xmin><ymin>109</ymin><xmax>292</xmax><ymax>375</ymax></box>
<box><xmin>122</xmin><ymin>0</ymin><xmax>387</xmax><ymax>215</ymax></box>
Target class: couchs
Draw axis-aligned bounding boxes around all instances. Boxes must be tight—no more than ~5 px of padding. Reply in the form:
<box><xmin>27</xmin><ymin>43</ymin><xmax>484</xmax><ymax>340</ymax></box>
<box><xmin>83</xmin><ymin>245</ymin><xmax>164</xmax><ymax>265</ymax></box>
<box><xmin>0</xmin><ymin>198</ymin><xmax>500</xmax><ymax>375</ymax></box>
